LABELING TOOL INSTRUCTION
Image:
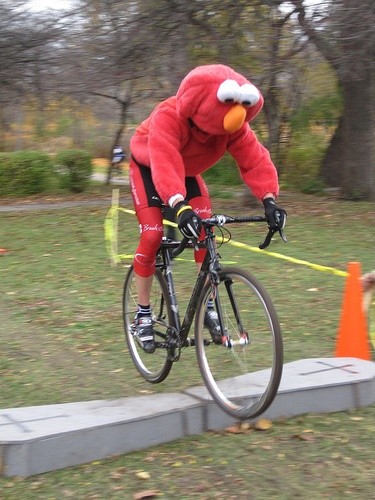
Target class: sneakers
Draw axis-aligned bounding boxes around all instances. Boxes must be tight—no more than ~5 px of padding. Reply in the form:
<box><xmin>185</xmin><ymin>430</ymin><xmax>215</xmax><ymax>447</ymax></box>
<box><xmin>131</xmin><ymin>311</ymin><xmax>156</xmax><ymax>352</ymax></box>
<box><xmin>204</xmin><ymin>309</ymin><xmax>228</xmax><ymax>345</ymax></box>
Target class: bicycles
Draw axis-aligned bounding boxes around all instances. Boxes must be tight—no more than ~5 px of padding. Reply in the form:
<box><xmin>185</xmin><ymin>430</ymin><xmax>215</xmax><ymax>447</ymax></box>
<box><xmin>122</xmin><ymin>211</ymin><xmax>288</xmax><ymax>420</ymax></box>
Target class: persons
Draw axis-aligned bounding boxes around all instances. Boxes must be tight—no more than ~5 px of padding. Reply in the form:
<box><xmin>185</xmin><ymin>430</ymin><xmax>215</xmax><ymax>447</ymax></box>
<box><xmin>127</xmin><ymin>64</ymin><xmax>286</xmax><ymax>352</ymax></box>
<box><xmin>111</xmin><ymin>142</ymin><xmax>125</xmax><ymax>178</ymax></box>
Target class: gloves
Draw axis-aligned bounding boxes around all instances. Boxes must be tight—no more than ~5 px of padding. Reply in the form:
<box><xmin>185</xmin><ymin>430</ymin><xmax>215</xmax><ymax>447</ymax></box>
<box><xmin>173</xmin><ymin>201</ymin><xmax>203</xmax><ymax>238</ymax></box>
<box><xmin>263</xmin><ymin>198</ymin><xmax>287</xmax><ymax>232</ymax></box>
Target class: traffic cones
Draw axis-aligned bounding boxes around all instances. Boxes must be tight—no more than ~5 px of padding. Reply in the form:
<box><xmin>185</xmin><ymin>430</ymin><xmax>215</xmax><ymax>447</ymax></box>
<box><xmin>334</xmin><ymin>262</ymin><xmax>373</xmax><ymax>363</ymax></box>
